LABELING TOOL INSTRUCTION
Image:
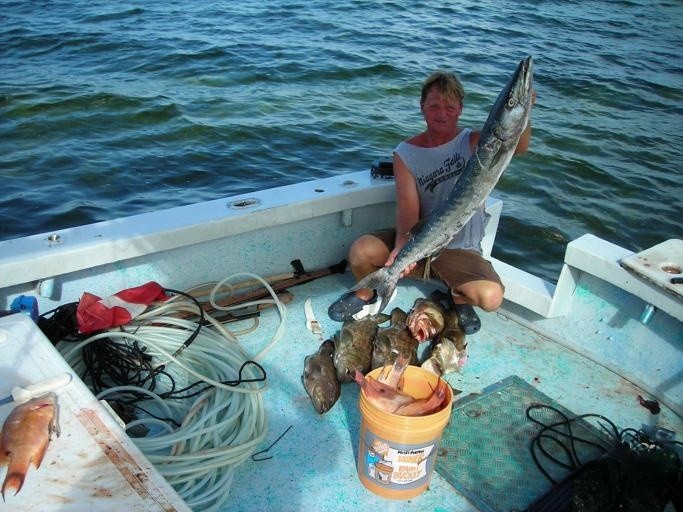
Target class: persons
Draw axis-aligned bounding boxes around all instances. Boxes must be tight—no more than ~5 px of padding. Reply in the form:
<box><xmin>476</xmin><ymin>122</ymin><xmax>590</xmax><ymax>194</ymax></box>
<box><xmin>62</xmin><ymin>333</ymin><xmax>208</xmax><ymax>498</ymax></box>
<box><xmin>328</xmin><ymin>70</ymin><xmax>536</xmax><ymax>334</ymax></box>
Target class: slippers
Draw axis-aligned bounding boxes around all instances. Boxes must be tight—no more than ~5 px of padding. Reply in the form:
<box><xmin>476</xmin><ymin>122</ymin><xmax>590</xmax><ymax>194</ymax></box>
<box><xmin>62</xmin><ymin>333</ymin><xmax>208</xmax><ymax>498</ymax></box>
<box><xmin>448</xmin><ymin>288</ymin><xmax>481</xmax><ymax>335</ymax></box>
<box><xmin>328</xmin><ymin>289</ymin><xmax>378</xmax><ymax>322</ymax></box>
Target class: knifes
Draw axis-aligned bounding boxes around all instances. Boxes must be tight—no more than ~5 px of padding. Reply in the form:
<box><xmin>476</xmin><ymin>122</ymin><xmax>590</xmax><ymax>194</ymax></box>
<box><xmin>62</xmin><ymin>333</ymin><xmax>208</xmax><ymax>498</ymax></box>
<box><xmin>0</xmin><ymin>372</ymin><xmax>71</xmax><ymax>405</ymax></box>
